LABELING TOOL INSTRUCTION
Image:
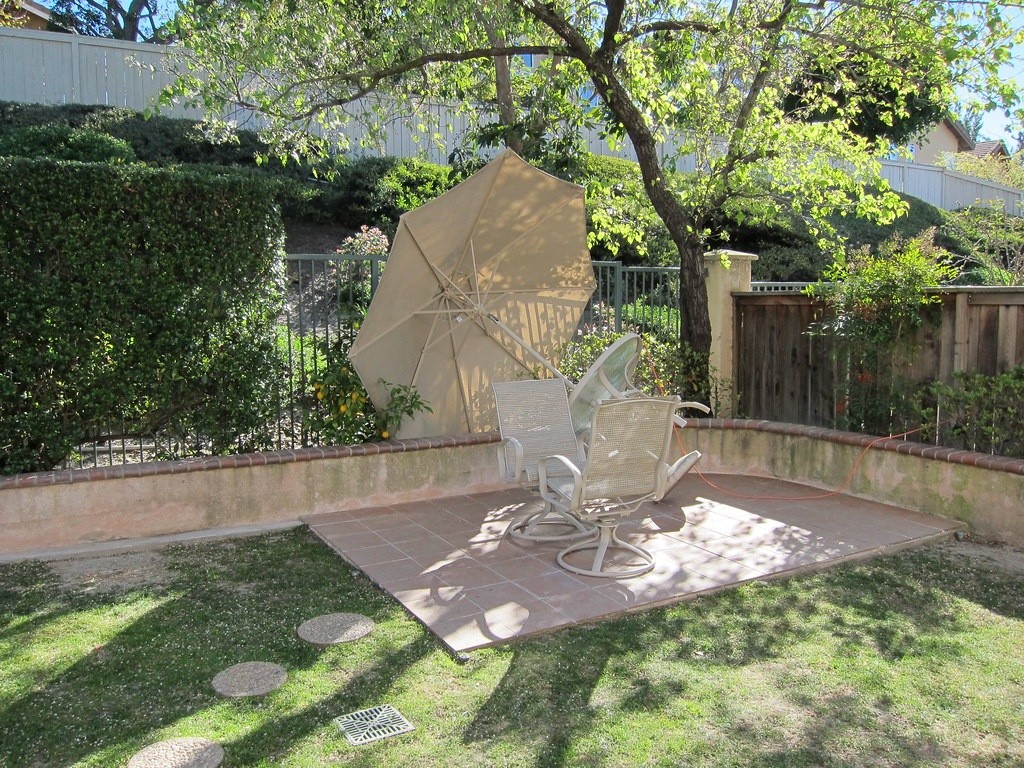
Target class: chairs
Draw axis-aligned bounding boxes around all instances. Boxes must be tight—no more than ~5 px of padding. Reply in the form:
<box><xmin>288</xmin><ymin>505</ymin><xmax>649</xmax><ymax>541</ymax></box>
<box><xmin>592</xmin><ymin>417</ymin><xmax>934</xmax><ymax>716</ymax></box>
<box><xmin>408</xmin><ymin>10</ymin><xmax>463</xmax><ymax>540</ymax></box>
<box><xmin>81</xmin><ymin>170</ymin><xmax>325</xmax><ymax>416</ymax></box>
<box><xmin>491</xmin><ymin>379</ymin><xmax>591</xmax><ymax>541</ymax></box>
<box><xmin>538</xmin><ymin>396</ymin><xmax>681</xmax><ymax>577</ymax></box>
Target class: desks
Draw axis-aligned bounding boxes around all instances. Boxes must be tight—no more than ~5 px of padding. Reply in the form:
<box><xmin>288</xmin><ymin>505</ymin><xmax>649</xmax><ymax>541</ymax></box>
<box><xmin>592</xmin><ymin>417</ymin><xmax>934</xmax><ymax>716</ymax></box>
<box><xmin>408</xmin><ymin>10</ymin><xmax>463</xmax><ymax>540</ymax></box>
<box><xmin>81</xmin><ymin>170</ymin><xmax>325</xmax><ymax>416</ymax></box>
<box><xmin>567</xmin><ymin>333</ymin><xmax>701</xmax><ymax>502</ymax></box>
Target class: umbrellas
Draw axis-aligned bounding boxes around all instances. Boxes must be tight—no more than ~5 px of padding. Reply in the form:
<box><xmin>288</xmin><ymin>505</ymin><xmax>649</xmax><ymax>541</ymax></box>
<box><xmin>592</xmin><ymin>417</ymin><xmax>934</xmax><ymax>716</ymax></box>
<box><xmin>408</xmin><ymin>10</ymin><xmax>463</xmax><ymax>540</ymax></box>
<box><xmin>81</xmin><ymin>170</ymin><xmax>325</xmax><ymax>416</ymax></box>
<box><xmin>346</xmin><ymin>146</ymin><xmax>672</xmax><ymax>472</ymax></box>
